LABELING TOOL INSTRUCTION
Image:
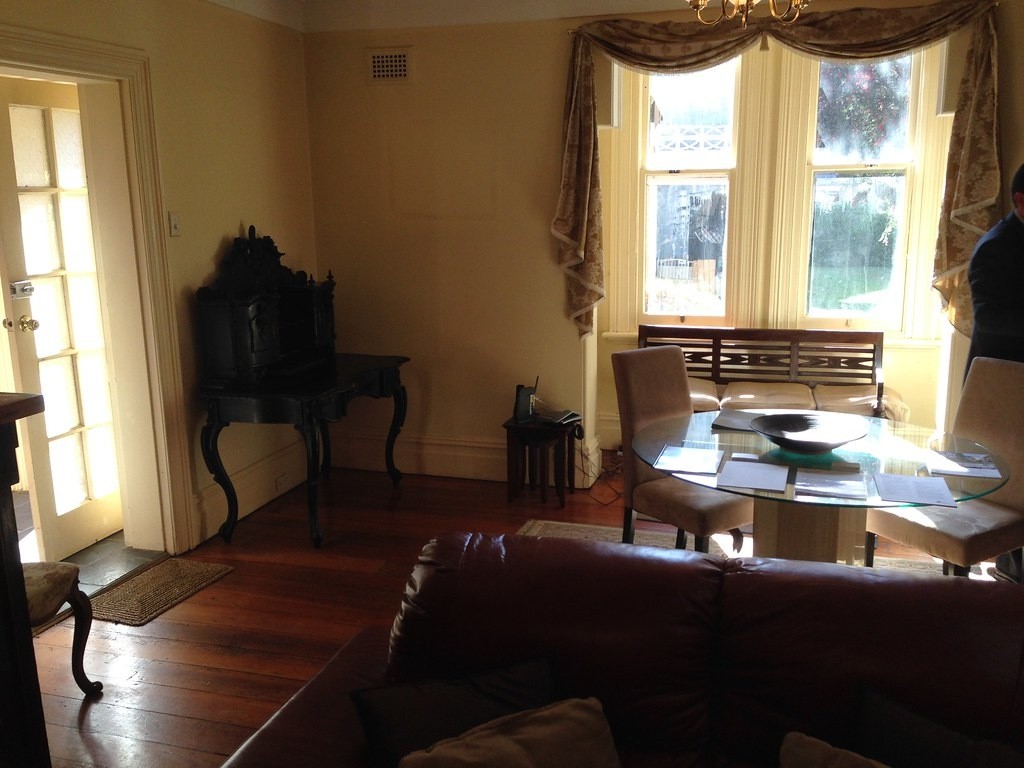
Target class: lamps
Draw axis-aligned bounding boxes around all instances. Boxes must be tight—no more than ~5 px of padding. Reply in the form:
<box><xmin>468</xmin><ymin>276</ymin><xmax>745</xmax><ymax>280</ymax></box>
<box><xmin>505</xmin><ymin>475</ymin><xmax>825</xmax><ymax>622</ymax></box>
<box><xmin>686</xmin><ymin>0</ymin><xmax>812</xmax><ymax>31</ymax></box>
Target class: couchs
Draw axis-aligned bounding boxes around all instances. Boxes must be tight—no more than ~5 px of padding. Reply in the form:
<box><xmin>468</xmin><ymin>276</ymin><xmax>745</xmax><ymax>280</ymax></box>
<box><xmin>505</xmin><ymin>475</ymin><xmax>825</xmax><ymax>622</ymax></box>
<box><xmin>215</xmin><ymin>530</ymin><xmax>1024</xmax><ymax>768</ymax></box>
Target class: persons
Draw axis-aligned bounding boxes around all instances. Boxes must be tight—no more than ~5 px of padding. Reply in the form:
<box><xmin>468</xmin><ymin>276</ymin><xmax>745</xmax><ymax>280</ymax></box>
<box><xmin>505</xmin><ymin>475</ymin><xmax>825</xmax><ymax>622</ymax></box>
<box><xmin>962</xmin><ymin>163</ymin><xmax>1024</xmax><ymax>394</ymax></box>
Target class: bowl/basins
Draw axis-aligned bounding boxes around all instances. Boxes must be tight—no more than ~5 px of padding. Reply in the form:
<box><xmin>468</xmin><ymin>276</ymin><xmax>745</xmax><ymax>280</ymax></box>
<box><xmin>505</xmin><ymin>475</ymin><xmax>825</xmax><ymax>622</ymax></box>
<box><xmin>749</xmin><ymin>412</ymin><xmax>869</xmax><ymax>455</ymax></box>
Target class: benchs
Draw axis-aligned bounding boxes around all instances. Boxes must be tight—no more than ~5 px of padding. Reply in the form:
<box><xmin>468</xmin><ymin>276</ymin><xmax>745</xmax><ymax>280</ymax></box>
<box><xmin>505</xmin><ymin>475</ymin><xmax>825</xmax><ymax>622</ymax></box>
<box><xmin>637</xmin><ymin>324</ymin><xmax>885</xmax><ymax>418</ymax></box>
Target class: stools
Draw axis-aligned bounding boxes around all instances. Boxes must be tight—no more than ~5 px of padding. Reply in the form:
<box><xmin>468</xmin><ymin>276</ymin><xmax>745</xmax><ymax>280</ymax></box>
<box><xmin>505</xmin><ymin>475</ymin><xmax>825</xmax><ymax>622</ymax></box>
<box><xmin>502</xmin><ymin>416</ymin><xmax>575</xmax><ymax>508</ymax></box>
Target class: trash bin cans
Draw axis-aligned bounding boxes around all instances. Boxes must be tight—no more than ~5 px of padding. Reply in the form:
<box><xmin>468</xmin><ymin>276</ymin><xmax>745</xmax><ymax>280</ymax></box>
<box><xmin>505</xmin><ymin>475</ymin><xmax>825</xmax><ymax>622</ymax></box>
<box><xmin>996</xmin><ymin>546</ymin><xmax>1024</xmax><ymax>582</ymax></box>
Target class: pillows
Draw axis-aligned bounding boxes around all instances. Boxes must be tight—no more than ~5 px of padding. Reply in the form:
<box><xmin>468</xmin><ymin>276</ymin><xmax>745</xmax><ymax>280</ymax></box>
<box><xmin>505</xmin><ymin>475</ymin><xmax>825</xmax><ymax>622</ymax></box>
<box><xmin>779</xmin><ymin>731</ymin><xmax>889</xmax><ymax>768</ymax></box>
<box><xmin>348</xmin><ymin>653</ymin><xmax>555</xmax><ymax>768</ymax></box>
<box><xmin>398</xmin><ymin>696</ymin><xmax>621</xmax><ymax>768</ymax></box>
<box><xmin>846</xmin><ymin>682</ymin><xmax>1024</xmax><ymax>768</ymax></box>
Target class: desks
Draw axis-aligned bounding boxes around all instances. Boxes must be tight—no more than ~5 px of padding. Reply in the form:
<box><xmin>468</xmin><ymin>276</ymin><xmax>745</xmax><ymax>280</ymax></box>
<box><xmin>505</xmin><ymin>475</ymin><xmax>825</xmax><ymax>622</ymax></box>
<box><xmin>196</xmin><ymin>353</ymin><xmax>411</xmax><ymax>551</ymax></box>
<box><xmin>0</xmin><ymin>393</ymin><xmax>53</xmax><ymax>768</ymax></box>
<box><xmin>630</xmin><ymin>408</ymin><xmax>1012</xmax><ymax>566</ymax></box>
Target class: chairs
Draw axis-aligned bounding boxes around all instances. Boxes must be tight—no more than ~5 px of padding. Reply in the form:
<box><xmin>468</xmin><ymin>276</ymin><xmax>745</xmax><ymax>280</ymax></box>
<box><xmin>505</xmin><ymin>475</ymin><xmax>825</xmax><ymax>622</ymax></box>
<box><xmin>611</xmin><ymin>344</ymin><xmax>754</xmax><ymax>552</ymax></box>
<box><xmin>864</xmin><ymin>357</ymin><xmax>1024</xmax><ymax>578</ymax></box>
<box><xmin>22</xmin><ymin>562</ymin><xmax>103</xmax><ymax>696</ymax></box>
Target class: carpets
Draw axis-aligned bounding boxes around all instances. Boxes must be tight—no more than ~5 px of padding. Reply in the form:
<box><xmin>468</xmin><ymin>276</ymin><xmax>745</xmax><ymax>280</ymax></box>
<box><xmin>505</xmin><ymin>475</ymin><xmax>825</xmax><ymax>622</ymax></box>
<box><xmin>515</xmin><ymin>519</ymin><xmax>996</xmax><ymax>580</ymax></box>
<box><xmin>90</xmin><ymin>557</ymin><xmax>235</xmax><ymax>627</ymax></box>
